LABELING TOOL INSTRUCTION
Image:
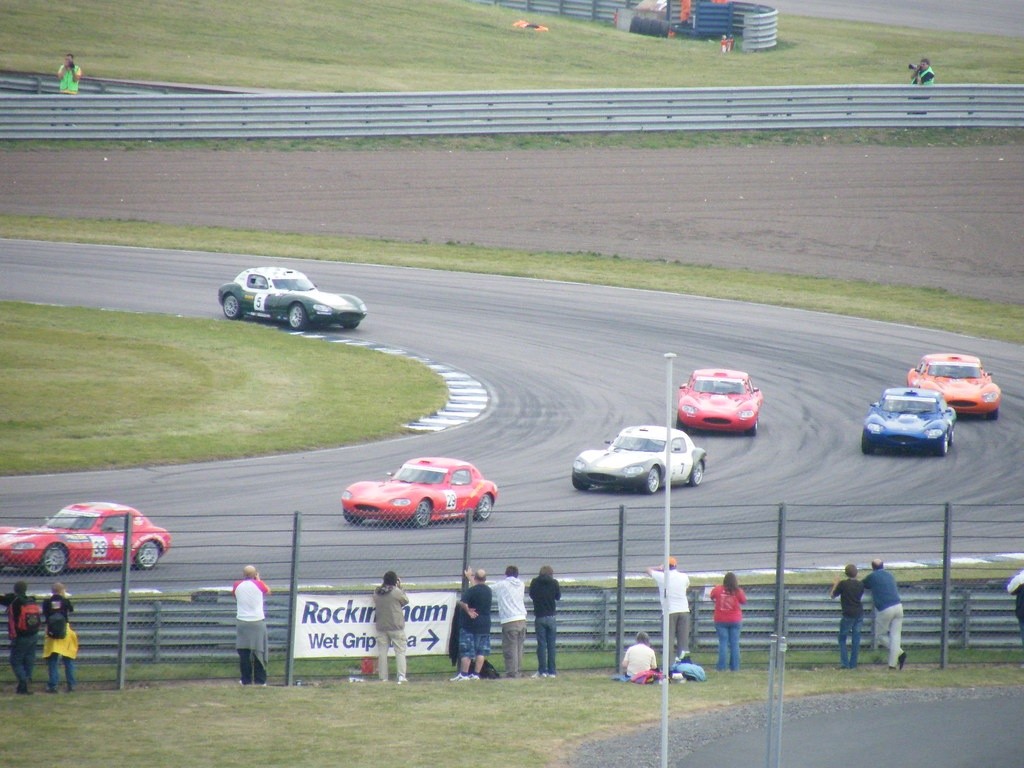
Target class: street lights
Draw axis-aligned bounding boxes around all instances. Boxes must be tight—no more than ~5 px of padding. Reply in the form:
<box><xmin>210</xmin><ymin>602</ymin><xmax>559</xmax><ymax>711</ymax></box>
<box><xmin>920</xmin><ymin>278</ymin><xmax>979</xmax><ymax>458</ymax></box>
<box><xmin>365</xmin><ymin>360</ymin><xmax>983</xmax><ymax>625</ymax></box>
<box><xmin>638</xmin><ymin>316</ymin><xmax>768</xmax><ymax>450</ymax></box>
<box><xmin>659</xmin><ymin>351</ymin><xmax>677</xmax><ymax>768</ymax></box>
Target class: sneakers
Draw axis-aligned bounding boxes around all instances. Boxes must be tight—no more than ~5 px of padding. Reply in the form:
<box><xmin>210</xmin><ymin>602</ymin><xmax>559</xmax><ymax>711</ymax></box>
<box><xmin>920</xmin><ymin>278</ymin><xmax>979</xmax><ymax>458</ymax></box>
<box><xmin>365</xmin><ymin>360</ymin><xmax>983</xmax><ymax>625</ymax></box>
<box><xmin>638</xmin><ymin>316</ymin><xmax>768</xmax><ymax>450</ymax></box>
<box><xmin>450</xmin><ymin>672</ymin><xmax>470</xmax><ymax>681</ymax></box>
<box><xmin>468</xmin><ymin>674</ymin><xmax>479</xmax><ymax>680</ymax></box>
<box><xmin>531</xmin><ymin>671</ymin><xmax>547</xmax><ymax>678</ymax></box>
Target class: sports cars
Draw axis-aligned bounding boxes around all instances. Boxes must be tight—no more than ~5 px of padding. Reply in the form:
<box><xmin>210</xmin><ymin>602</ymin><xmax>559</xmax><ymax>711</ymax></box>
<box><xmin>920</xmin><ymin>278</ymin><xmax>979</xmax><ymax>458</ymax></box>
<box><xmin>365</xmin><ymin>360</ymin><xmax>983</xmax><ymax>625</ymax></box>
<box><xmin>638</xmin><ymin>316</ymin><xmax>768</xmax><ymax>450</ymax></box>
<box><xmin>677</xmin><ymin>368</ymin><xmax>765</xmax><ymax>436</ymax></box>
<box><xmin>340</xmin><ymin>457</ymin><xmax>499</xmax><ymax>529</ymax></box>
<box><xmin>906</xmin><ymin>353</ymin><xmax>1003</xmax><ymax>423</ymax></box>
<box><xmin>859</xmin><ymin>387</ymin><xmax>958</xmax><ymax>455</ymax></box>
<box><xmin>571</xmin><ymin>424</ymin><xmax>708</xmax><ymax>494</ymax></box>
<box><xmin>0</xmin><ymin>502</ymin><xmax>172</xmax><ymax>572</ymax></box>
<box><xmin>218</xmin><ymin>266</ymin><xmax>368</xmax><ymax>333</ymax></box>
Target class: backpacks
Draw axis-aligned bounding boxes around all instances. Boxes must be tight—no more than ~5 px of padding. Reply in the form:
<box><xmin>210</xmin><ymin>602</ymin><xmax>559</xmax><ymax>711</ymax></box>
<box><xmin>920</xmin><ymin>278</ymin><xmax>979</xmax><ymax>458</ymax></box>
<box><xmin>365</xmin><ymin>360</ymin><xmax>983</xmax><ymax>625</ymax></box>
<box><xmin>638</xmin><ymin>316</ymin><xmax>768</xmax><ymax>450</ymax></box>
<box><xmin>46</xmin><ymin>612</ymin><xmax>66</xmax><ymax>639</ymax></box>
<box><xmin>13</xmin><ymin>596</ymin><xmax>40</xmax><ymax>635</ymax></box>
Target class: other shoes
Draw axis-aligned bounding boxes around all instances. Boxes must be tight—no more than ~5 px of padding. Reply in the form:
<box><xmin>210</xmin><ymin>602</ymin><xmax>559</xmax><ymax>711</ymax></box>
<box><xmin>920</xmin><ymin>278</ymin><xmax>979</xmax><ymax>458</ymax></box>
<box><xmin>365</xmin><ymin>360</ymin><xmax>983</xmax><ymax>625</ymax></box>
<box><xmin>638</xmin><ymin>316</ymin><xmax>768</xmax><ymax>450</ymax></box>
<box><xmin>68</xmin><ymin>684</ymin><xmax>75</xmax><ymax>692</ymax></box>
<box><xmin>547</xmin><ymin>673</ymin><xmax>555</xmax><ymax>678</ymax></box>
<box><xmin>669</xmin><ymin>556</ymin><xmax>677</xmax><ymax>566</ymax></box>
<box><xmin>25</xmin><ymin>676</ymin><xmax>34</xmax><ymax>694</ymax></box>
<box><xmin>46</xmin><ymin>686</ymin><xmax>57</xmax><ymax>693</ymax></box>
<box><xmin>17</xmin><ymin>683</ymin><xmax>25</xmax><ymax>693</ymax></box>
<box><xmin>899</xmin><ymin>652</ymin><xmax>906</xmax><ymax>670</ymax></box>
<box><xmin>833</xmin><ymin>666</ymin><xmax>847</xmax><ymax>670</ymax></box>
<box><xmin>397</xmin><ymin>677</ymin><xmax>407</xmax><ymax>682</ymax></box>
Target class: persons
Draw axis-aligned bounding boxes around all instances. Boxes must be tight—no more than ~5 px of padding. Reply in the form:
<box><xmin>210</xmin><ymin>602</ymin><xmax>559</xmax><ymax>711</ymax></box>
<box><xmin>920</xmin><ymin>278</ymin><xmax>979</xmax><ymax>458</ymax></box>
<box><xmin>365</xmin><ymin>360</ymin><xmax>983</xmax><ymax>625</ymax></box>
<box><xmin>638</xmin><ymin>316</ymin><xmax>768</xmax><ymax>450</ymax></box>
<box><xmin>231</xmin><ymin>565</ymin><xmax>272</xmax><ymax>687</ymax></box>
<box><xmin>57</xmin><ymin>52</ymin><xmax>82</xmax><ymax>127</ymax></box>
<box><xmin>860</xmin><ymin>559</ymin><xmax>907</xmax><ymax>670</ymax></box>
<box><xmin>4</xmin><ymin>581</ymin><xmax>41</xmax><ymax>695</ymax></box>
<box><xmin>905</xmin><ymin>59</ymin><xmax>935</xmax><ymax>129</ymax></box>
<box><xmin>710</xmin><ymin>572</ymin><xmax>747</xmax><ymax>672</ymax></box>
<box><xmin>374</xmin><ymin>570</ymin><xmax>410</xmax><ymax>681</ymax></box>
<box><xmin>530</xmin><ymin>565</ymin><xmax>561</xmax><ymax>677</ymax></box>
<box><xmin>1007</xmin><ymin>569</ymin><xmax>1024</xmax><ymax>670</ymax></box>
<box><xmin>645</xmin><ymin>554</ymin><xmax>691</xmax><ymax>665</ymax></box>
<box><xmin>449</xmin><ymin>565</ymin><xmax>492</xmax><ymax>681</ymax></box>
<box><xmin>491</xmin><ymin>565</ymin><xmax>527</xmax><ymax>677</ymax></box>
<box><xmin>830</xmin><ymin>564</ymin><xmax>865</xmax><ymax>669</ymax></box>
<box><xmin>622</xmin><ymin>630</ymin><xmax>659</xmax><ymax>678</ymax></box>
<box><xmin>43</xmin><ymin>582</ymin><xmax>78</xmax><ymax>693</ymax></box>
